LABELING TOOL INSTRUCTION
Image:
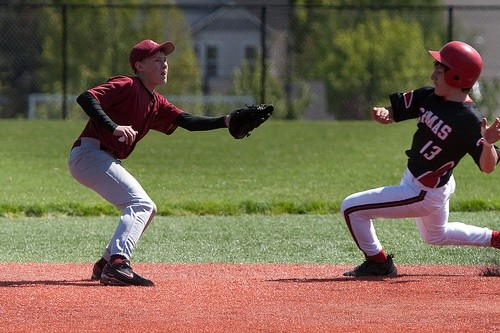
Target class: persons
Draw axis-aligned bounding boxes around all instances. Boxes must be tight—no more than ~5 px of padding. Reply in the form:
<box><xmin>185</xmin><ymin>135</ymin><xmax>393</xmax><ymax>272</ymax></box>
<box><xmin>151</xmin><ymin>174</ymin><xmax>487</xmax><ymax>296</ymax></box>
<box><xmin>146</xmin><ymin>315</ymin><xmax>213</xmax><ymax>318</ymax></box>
<box><xmin>69</xmin><ymin>39</ymin><xmax>230</xmax><ymax>286</ymax></box>
<box><xmin>341</xmin><ymin>40</ymin><xmax>500</xmax><ymax>278</ymax></box>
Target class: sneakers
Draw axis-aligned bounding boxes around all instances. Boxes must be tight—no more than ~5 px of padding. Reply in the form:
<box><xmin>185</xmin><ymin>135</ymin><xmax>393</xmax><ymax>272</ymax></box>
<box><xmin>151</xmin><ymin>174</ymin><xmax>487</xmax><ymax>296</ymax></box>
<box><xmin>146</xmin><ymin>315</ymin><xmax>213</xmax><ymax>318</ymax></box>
<box><xmin>343</xmin><ymin>253</ymin><xmax>397</xmax><ymax>276</ymax></box>
<box><xmin>100</xmin><ymin>259</ymin><xmax>154</xmax><ymax>285</ymax></box>
<box><xmin>91</xmin><ymin>260</ymin><xmax>104</xmax><ymax>280</ymax></box>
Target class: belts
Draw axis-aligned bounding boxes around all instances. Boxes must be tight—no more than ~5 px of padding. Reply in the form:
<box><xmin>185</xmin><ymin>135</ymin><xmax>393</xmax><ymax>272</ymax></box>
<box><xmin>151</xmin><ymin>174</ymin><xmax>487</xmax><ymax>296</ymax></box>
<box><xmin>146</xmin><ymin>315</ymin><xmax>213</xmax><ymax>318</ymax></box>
<box><xmin>71</xmin><ymin>139</ymin><xmax>106</xmax><ymax>150</ymax></box>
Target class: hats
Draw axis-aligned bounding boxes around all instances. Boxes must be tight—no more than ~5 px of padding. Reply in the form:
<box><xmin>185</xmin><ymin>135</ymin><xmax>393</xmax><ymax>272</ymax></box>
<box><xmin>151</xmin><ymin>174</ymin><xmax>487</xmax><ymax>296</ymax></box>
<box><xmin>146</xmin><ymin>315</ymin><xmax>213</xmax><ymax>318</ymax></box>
<box><xmin>130</xmin><ymin>38</ymin><xmax>175</xmax><ymax>74</ymax></box>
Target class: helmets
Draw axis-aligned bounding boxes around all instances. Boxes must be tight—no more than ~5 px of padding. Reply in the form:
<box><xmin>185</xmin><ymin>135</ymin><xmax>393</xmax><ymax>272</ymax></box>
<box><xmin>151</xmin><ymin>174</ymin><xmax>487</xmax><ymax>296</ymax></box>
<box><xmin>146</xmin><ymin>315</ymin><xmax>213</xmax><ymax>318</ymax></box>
<box><xmin>428</xmin><ymin>40</ymin><xmax>483</xmax><ymax>88</ymax></box>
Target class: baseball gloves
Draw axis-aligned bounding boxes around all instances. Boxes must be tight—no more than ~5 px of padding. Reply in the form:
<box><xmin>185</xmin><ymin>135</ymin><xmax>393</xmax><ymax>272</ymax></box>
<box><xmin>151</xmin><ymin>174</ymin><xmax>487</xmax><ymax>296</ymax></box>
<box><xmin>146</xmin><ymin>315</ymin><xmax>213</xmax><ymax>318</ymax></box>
<box><xmin>229</xmin><ymin>104</ymin><xmax>274</xmax><ymax>139</ymax></box>
